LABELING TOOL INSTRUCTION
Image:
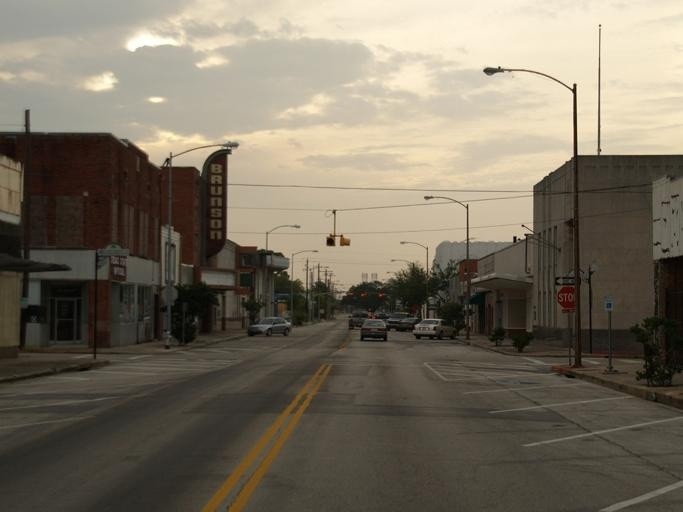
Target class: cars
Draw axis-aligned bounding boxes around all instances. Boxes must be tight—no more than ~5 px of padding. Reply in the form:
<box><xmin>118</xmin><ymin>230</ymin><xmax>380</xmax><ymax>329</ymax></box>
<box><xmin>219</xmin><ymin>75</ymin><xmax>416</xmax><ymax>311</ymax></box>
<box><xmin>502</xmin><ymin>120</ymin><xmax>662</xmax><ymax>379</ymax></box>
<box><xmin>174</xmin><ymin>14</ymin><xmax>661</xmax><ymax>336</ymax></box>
<box><xmin>348</xmin><ymin>310</ymin><xmax>456</xmax><ymax>342</ymax></box>
<box><xmin>246</xmin><ymin>317</ymin><xmax>290</xmax><ymax>338</ymax></box>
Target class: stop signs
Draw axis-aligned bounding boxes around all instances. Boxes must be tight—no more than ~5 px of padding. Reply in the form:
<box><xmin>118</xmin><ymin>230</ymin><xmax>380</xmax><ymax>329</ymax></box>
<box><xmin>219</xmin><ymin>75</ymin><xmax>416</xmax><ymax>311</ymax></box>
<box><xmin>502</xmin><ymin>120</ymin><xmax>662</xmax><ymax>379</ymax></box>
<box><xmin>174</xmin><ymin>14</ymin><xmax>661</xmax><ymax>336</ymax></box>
<box><xmin>556</xmin><ymin>286</ymin><xmax>576</xmax><ymax>308</ymax></box>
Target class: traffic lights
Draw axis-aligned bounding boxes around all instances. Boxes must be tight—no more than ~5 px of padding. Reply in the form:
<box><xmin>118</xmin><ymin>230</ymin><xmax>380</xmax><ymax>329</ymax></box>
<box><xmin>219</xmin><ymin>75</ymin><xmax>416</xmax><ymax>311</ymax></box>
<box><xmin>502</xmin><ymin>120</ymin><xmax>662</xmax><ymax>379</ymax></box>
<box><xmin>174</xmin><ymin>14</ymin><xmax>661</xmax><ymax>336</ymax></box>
<box><xmin>325</xmin><ymin>237</ymin><xmax>335</xmax><ymax>246</ymax></box>
<box><xmin>339</xmin><ymin>236</ymin><xmax>351</xmax><ymax>246</ymax></box>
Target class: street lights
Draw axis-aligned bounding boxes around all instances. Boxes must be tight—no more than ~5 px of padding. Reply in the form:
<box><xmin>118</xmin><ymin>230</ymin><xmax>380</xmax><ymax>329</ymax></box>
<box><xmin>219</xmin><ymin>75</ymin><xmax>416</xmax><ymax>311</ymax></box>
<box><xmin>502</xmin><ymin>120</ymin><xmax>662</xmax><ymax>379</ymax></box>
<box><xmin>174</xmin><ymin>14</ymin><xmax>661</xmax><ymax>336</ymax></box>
<box><xmin>264</xmin><ymin>224</ymin><xmax>300</xmax><ymax>318</ymax></box>
<box><xmin>391</xmin><ymin>259</ymin><xmax>414</xmax><ymax>317</ymax></box>
<box><xmin>483</xmin><ymin>65</ymin><xmax>587</xmax><ymax>366</ymax></box>
<box><xmin>399</xmin><ymin>240</ymin><xmax>429</xmax><ymax>319</ymax></box>
<box><xmin>288</xmin><ymin>249</ymin><xmax>319</xmax><ymax>322</ymax></box>
<box><xmin>308</xmin><ymin>265</ymin><xmax>343</xmax><ymax>320</ymax></box>
<box><xmin>424</xmin><ymin>196</ymin><xmax>471</xmax><ymax>338</ymax></box>
<box><xmin>160</xmin><ymin>141</ymin><xmax>238</xmax><ymax>348</ymax></box>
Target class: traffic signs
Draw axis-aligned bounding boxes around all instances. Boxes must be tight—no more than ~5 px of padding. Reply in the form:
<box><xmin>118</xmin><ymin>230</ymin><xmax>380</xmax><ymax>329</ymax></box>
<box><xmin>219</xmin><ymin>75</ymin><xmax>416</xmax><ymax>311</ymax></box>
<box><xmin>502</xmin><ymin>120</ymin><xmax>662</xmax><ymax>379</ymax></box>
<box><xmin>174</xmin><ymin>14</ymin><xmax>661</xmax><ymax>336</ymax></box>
<box><xmin>554</xmin><ymin>276</ymin><xmax>581</xmax><ymax>286</ymax></box>
